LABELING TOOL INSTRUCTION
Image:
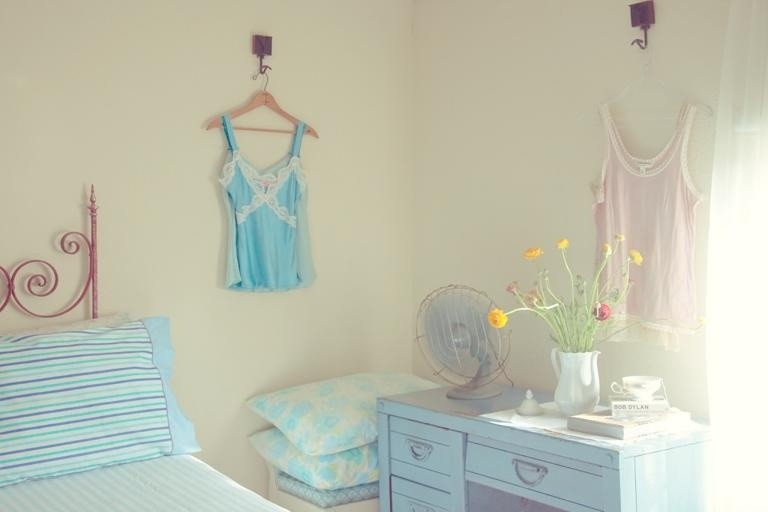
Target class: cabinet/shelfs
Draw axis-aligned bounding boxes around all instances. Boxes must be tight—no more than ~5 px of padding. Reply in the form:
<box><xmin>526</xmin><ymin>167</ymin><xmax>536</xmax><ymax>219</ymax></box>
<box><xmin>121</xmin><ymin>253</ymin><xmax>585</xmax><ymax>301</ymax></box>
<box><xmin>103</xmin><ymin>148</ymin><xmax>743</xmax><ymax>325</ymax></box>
<box><xmin>378</xmin><ymin>388</ymin><xmax>707</xmax><ymax>510</ymax></box>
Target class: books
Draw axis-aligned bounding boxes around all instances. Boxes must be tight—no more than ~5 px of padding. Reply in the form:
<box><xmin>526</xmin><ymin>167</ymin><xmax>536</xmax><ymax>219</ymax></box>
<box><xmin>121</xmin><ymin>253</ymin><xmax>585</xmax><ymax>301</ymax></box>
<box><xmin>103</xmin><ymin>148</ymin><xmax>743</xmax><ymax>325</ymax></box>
<box><xmin>565</xmin><ymin>408</ymin><xmax>696</xmax><ymax>440</ymax></box>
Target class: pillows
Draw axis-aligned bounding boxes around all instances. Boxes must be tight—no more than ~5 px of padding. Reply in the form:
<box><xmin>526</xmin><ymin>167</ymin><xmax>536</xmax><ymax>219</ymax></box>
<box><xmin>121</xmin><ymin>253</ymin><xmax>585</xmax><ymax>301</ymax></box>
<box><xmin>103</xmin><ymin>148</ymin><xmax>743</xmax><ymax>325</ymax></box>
<box><xmin>0</xmin><ymin>314</ymin><xmax>200</xmax><ymax>485</ymax></box>
<box><xmin>246</xmin><ymin>367</ymin><xmax>433</xmax><ymax>507</ymax></box>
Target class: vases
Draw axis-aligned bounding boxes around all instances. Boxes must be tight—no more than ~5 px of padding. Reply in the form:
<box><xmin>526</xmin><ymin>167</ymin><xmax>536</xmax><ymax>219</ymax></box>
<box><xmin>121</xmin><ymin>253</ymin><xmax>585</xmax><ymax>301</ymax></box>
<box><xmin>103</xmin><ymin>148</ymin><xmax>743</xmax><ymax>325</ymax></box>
<box><xmin>553</xmin><ymin>340</ymin><xmax>604</xmax><ymax>417</ymax></box>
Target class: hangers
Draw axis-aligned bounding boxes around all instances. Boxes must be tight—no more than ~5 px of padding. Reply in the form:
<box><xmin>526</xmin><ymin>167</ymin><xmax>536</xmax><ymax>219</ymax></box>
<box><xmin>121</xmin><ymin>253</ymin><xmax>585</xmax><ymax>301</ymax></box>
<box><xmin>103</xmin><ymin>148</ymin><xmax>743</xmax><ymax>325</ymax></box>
<box><xmin>201</xmin><ymin>72</ymin><xmax>325</xmax><ymax>136</ymax></box>
<box><xmin>594</xmin><ymin>46</ymin><xmax>714</xmax><ymax>125</ymax></box>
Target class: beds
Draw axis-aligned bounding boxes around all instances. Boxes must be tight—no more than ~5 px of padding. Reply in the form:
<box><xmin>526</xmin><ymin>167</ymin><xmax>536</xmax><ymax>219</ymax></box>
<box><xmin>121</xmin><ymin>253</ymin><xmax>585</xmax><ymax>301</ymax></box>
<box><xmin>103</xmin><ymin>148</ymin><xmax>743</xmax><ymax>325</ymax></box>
<box><xmin>0</xmin><ymin>182</ymin><xmax>293</xmax><ymax>510</ymax></box>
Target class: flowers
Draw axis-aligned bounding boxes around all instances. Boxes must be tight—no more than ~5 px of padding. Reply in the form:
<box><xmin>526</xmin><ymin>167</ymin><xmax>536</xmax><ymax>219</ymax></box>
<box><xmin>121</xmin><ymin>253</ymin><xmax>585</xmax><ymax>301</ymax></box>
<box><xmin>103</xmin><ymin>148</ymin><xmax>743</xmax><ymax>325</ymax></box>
<box><xmin>481</xmin><ymin>231</ymin><xmax>656</xmax><ymax>356</ymax></box>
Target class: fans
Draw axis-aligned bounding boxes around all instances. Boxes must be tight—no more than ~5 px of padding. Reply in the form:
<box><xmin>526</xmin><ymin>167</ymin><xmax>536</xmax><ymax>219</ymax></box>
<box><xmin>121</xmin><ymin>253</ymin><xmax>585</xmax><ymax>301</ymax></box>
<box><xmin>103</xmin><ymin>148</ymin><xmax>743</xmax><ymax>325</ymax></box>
<box><xmin>414</xmin><ymin>285</ymin><xmax>518</xmax><ymax>403</ymax></box>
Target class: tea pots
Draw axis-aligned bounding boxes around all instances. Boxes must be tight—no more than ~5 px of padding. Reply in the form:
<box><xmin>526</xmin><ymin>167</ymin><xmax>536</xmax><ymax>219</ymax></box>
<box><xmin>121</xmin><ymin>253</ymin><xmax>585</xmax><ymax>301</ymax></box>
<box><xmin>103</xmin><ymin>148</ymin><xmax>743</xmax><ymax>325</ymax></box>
<box><xmin>549</xmin><ymin>348</ymin><xmax>601</xmax><ymax>418</ymax></box>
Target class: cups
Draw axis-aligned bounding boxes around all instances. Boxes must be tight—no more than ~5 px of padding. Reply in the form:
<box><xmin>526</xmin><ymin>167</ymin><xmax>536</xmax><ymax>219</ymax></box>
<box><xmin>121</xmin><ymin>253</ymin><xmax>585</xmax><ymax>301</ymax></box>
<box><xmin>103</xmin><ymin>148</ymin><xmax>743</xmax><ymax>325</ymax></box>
<box><xmin>611</xmin><ymin>376</ymin><xmax>662</xmax><ymax>400</ymax></box>
